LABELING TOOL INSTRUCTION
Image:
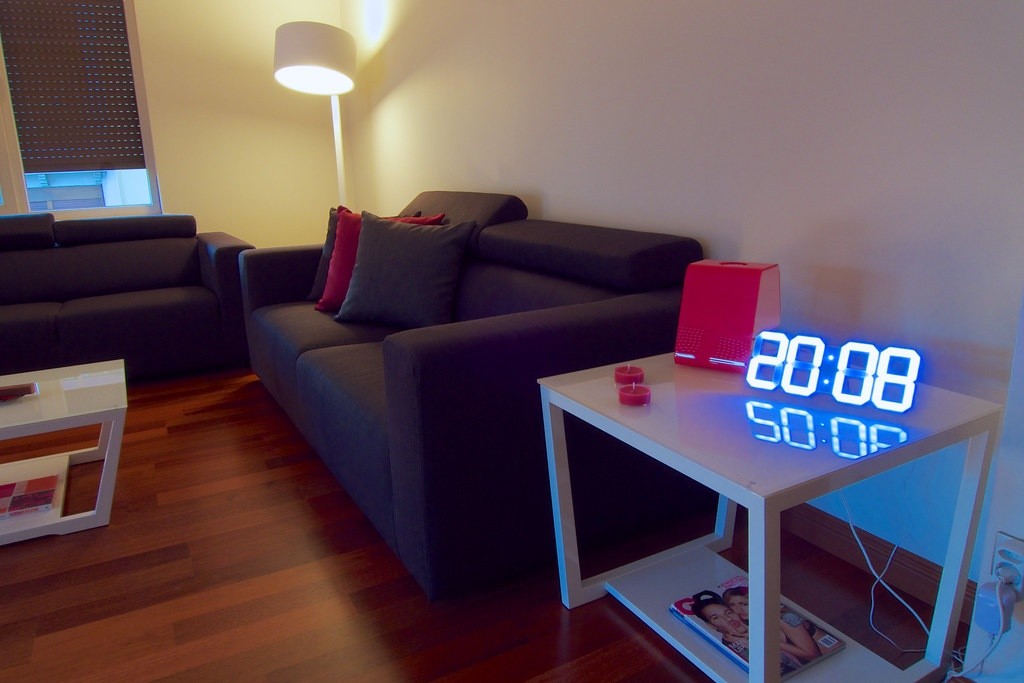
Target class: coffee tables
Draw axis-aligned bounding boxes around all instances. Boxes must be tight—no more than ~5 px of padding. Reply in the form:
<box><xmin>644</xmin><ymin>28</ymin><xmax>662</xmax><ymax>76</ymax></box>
<box><xmin>0</xmin><ymin>358</ymin><xmax>129</xmax><ymax>547</ymax></box>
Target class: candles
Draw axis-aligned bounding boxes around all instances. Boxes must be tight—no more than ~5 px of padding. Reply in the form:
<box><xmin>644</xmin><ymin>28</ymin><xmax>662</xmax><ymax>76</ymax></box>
<box><xmin>619</xmin><ymin>380</ymin><xmax>651</xmax><ymax>407</ymax></box>
<box><xmin>614</xmin><ymin>362</ymin><xmax>644</xmax><ymax>384</ymax></box>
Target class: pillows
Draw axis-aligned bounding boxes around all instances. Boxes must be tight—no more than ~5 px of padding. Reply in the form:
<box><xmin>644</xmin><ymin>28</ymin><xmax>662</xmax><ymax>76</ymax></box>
<box><xmin>332</xmin><ymin>210</ymin><xmax>476</xmax><ymax>329</ymax></box>
<box><xmin>314</xmin><ymin>204</ymin><xmax>446</xmax><ymax>313</ymax></box>
<box><xmin>304</xmin><ymin>207</ymin><xmax>422</xmax><ymax>302</ymax></box>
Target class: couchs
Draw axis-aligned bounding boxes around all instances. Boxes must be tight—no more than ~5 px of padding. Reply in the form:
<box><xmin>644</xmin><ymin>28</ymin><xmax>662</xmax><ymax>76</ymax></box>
<box><xmin>237</xmin><ymin>191</ymin><xmax>713</xmax><ymax>604</ymax></box>
<box><xmin>0</xmin><ymin>213</ymin><xmax>256</xmax><ymax>383</ymax></box>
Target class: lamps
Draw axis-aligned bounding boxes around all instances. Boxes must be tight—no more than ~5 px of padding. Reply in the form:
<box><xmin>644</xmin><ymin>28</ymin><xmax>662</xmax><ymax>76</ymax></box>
<box><xmin>269</xmin><ymin>18</ymin><xmax>357</xmax><ymax>205</ymax></box>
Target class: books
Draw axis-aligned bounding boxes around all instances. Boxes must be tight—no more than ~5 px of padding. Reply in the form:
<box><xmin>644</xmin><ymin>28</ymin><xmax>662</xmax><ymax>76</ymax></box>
<box><xmin>0</xmin><ymin>474</ymin><xmax>60</xmax><ymax>521</ymax></box>
<box><xmin>668</xmin><ymin>572</ymin><xmax>847</xmax><ymax>682</ymax></box>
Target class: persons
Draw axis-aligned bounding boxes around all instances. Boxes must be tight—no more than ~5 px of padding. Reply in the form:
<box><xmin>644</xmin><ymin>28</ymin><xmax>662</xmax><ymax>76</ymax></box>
<box><xmin>691</xmin><ymin>586</ymin><xmax>843</xmax><ymax>676</ymax></box>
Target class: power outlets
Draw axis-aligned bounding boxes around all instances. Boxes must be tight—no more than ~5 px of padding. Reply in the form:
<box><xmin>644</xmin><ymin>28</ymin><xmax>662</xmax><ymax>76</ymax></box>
<box><xmin>986</xmin><ymin>529</ymin><xmax>1024</xmax><ymax>602</ymax></box>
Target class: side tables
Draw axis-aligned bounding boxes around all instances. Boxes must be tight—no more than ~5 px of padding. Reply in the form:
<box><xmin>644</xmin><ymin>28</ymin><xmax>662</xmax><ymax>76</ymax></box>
<box><xmin>537</xmin><ymin>350</ymin><xmax>1004</xmax><ymax>683</ymax></box>
